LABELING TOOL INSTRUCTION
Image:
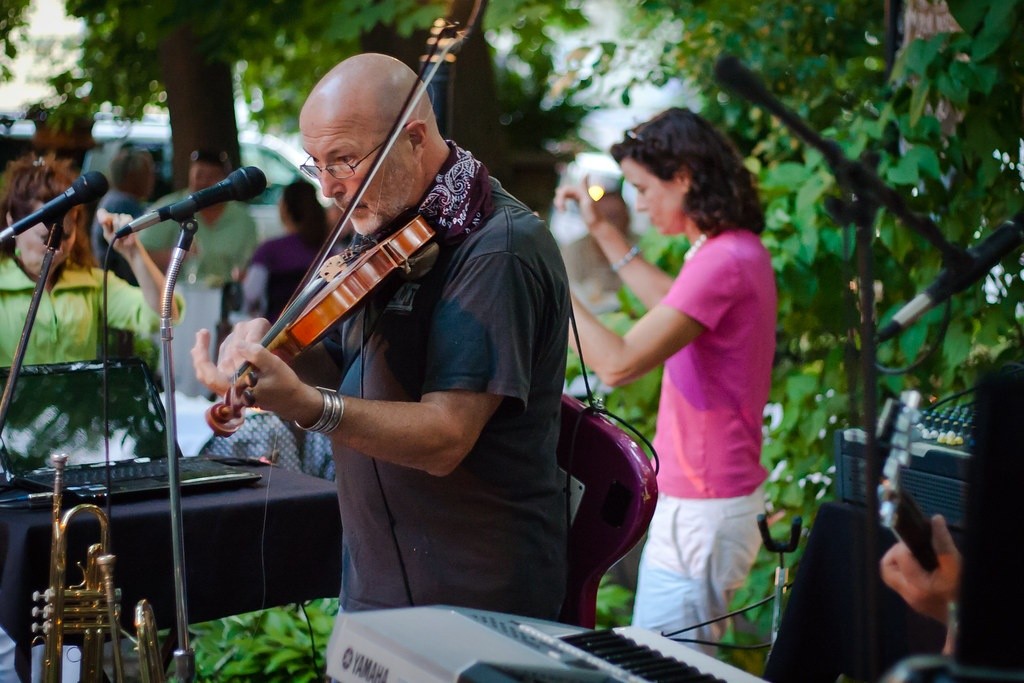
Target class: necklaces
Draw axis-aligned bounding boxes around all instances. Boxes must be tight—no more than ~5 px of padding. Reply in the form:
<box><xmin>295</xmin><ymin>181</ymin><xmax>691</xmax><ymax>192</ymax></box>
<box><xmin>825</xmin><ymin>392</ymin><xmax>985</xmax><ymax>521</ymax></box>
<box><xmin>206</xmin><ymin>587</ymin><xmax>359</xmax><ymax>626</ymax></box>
<box><xmin>684</xmin><ymin>229</ymin><xmax>714</xmax><ymax>260</ymax></box>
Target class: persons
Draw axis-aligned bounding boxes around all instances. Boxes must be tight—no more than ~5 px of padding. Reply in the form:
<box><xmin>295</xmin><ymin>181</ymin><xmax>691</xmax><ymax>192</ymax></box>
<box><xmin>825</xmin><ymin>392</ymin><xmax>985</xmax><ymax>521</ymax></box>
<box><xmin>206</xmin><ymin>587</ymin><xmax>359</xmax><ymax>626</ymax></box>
<box><xmin>879</xmin><ymin>345</ymin><xmax>1024</xmax><ymax>681</ymax></box>
<box><xmin>552</xmin><ymin>107</ymin><xmax>778</xmax><ymax>658</ymax></box>
<box><xmin>0</xmin><ymin>145</ymin><xmax>357</xmax><ymax>400</ymax></box>
<box><xmin>565</xmin><ymin>190</ymin><xmax>641</xmax><ymax>294</ymax></box>
<box><xmin>189</xmin><ymin>53</ymin><xmax>571</xmax><ymax>620</ymax></box>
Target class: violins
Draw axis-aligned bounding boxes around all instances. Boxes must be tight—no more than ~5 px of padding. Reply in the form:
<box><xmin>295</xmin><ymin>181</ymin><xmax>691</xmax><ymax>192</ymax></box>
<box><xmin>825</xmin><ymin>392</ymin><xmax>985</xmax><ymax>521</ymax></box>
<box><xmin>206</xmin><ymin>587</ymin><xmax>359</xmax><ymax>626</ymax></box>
<box><xmin>201</xmin><ymin>216</ymin><xmax>437</xmax><ymax>437</ymax></box>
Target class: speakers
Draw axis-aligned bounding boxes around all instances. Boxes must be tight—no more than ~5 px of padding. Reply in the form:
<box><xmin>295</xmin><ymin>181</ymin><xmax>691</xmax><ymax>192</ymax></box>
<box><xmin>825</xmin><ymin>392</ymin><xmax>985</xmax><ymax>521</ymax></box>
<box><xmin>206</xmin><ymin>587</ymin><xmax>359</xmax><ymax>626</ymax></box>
<box><xmin>833</xmin><ymin>426</ymin><xmax>976</xmax><ymax>532</ymax></box>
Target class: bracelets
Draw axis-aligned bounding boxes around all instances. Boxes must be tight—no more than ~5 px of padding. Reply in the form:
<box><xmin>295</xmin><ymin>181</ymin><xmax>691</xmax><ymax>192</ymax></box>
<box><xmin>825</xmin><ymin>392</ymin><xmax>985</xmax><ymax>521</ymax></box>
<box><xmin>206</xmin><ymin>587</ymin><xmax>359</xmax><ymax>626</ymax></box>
<box><xmin>613</xmin><ymin>247</ymin><xmax>641</xmax><ymax>271</ymax></box>
<box><xmin>295</xmin><ymin>387</ymin><xmax>344</xmax><ymax>434</ymax></box>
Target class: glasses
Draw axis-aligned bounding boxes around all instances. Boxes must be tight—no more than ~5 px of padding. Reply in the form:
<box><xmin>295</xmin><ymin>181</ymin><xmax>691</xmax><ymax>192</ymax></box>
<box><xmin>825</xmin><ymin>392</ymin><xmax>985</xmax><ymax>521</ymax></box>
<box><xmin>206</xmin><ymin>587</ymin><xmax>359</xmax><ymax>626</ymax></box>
<box><xmin>622</xmin><ymin>127</ymin><xmax>695</xmax><ymax>173</ymax></box>
<box><xmin>299</xmin><ymin>123</ymin><xmax>410</xmax><ymax>180</ymax></box>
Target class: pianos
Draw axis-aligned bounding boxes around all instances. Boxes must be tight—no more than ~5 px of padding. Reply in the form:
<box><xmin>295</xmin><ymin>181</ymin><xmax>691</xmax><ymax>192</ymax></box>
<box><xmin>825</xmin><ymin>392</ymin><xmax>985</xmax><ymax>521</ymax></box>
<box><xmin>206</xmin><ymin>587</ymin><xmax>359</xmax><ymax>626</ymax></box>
<box><xmin>323</xmin><ymin>602</ymin><xmax>781</xmax><ymax>683</ymax></box>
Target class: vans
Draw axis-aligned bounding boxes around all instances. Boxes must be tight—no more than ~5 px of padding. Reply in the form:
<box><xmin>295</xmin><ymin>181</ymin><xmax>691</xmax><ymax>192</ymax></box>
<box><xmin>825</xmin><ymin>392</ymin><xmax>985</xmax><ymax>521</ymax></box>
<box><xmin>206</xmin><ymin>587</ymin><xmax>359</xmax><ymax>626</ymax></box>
<box><xmin>0</xmin><ymin>111</ymin><xmax>339</xmax><ymax>247</ymax></box>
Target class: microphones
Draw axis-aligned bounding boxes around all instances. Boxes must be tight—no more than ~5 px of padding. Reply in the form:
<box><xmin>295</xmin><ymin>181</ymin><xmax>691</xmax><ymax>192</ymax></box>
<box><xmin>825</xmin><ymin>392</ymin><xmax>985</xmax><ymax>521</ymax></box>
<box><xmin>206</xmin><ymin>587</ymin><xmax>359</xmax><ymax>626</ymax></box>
<box><xmin>114</xmin><ymin>164</ymin><xmax>267</xmax><ymax>242</ymax></box>
<box><xmin>0</xmin><ymin>170</ymin><xmax>110</xmax><ymax>245</ymax></box>
<box><xmin>875</xmin><ymin>205</ymin><xmax>1024</xmax><ymax>343</ymax></box>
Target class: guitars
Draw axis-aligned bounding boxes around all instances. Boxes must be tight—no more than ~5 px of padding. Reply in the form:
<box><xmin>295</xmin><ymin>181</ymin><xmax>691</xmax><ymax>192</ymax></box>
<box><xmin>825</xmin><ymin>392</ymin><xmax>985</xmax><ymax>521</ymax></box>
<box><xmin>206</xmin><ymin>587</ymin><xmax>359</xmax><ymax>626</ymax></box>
<box><xmin>556</xmin><ymin>394</ymin><xmax>657</xmax><ymax>628</ymax></box>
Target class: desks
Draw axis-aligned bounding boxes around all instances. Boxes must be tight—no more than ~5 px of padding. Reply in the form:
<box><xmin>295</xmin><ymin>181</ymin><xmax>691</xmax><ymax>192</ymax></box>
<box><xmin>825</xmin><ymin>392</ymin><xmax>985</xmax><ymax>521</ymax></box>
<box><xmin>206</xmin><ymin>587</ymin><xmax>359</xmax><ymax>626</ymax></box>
<box><xmin>0</xmin><ymin>459</ymin><xmax>344</xmax><ymax>683</ymax></box>
<box><xmin>763</xmin><ymin>500</ymin><xmax>954</xmax><ymax>683</ymax></box>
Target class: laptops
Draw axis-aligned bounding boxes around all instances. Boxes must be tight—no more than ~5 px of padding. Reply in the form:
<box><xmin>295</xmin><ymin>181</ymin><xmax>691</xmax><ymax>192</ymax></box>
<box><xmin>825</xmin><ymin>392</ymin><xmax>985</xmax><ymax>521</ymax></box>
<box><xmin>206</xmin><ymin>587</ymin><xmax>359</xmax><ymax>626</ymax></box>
<box><xmin>0</xmin><ymin>356</ymin><xmax>263</xmax><ymax>505</ymax></box>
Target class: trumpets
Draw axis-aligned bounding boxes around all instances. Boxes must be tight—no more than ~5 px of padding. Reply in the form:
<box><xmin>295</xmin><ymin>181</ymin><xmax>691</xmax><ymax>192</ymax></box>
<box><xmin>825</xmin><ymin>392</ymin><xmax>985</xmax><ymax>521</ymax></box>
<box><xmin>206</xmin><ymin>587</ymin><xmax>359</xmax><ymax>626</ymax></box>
<box><xmin>25</xmin><ymin>452</ymin><xmax>167</xmax><ymax>683</ymax></box>
